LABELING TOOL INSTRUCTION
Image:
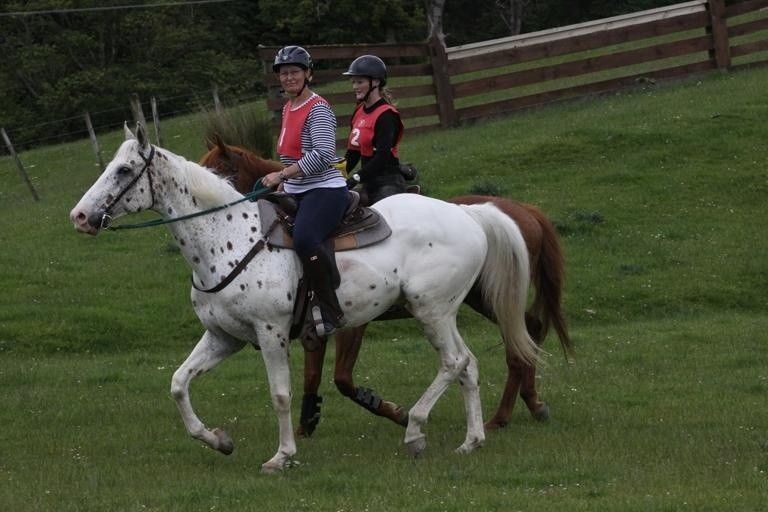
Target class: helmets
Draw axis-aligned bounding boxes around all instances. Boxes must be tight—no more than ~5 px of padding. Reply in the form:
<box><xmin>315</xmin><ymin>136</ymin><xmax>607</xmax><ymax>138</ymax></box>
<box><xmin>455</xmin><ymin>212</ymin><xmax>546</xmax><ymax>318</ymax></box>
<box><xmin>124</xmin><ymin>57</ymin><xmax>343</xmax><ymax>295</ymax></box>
<box><xmin>273</xmin><ymin>45</ymin><xmax>313</xmax><ymax>68</ymax></box>
<box><xmin>342</xmin><ymin>54</ymin><xmax>388</xmax><ymax>78</ymax></box>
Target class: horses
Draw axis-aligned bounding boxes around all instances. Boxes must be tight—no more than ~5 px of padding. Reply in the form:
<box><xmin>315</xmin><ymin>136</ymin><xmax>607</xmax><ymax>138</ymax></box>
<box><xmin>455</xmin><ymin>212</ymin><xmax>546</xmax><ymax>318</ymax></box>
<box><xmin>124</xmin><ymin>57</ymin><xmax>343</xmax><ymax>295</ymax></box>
<box><xmin>196</xmin><ymin>104</ymin><xmax>575</xmax><ymax>444</ymax></box>
<box><xmin>67</xmin><ymin>119</ymin><xmax>553</xmax><ymax>472</ymax></box>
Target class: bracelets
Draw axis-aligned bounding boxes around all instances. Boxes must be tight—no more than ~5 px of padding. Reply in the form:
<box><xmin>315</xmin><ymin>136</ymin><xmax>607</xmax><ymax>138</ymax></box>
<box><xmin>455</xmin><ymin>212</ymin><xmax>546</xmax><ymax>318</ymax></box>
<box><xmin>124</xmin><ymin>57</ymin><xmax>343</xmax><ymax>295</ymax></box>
<box><xmin>278</xmin><ymin>169</ymin><xmax>289</xmax><ymax>182</ymax></box>
<box><xmin>353</xmin><ymin>173</ymin><xmax>361</xmax><ymax>182</ymax></box>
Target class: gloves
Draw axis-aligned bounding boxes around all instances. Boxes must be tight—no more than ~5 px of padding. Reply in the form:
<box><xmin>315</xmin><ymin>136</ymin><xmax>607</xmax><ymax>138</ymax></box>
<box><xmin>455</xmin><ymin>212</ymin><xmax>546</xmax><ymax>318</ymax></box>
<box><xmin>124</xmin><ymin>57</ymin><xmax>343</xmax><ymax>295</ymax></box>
<box><xmin>346</xmin><ymin>173</ymin><xmax>360</xmax><ymax>191</ymax></box>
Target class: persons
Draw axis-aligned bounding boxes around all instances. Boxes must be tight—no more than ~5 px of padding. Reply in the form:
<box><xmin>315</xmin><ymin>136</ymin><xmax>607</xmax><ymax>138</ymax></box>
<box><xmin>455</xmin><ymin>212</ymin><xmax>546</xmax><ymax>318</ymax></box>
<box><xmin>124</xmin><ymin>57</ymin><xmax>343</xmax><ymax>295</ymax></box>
<box><xmin>341</xmin><ymin>53</ymin><xmax>407</xmax><ymax>203</ymax></box>
<box><xmin>260</xmin><ymin>44</ymin><xmax>353</xmax><ymax>335</ymax></box>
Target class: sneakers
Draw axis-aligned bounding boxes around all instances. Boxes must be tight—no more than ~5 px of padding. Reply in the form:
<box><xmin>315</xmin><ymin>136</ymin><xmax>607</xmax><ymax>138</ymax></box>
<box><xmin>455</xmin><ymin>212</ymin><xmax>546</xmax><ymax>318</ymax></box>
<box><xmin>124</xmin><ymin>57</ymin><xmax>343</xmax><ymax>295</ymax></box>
<box><xmin>309</xmin><ymin>314</ymin><xmax>347</xmax><ymax>337</ymax></box>
